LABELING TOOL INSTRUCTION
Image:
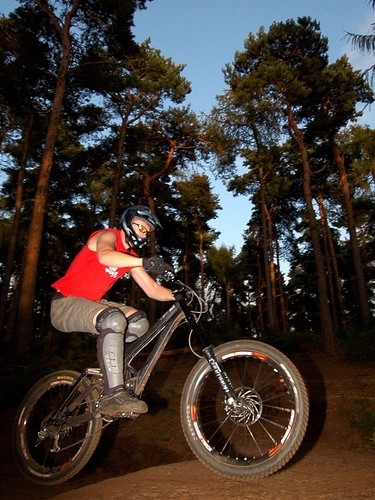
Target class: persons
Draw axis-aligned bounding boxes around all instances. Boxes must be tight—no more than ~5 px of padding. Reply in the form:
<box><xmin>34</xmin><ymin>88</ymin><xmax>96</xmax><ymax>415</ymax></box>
<box><xmin>50</xmin><ymin>205</ymin><xmax>192</xmax><ymax>417</ymax></box>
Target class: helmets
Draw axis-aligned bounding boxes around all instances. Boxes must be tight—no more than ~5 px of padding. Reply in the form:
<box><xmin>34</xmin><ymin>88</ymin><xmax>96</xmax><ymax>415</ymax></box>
<box><xmin>120</xmin><ymin>204</ymin><xmax>162</xmax><ymax>255</ymax></box>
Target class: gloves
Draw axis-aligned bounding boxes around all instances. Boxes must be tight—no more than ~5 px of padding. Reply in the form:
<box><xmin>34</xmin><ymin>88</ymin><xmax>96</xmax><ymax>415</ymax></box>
<box><xmin>143</xmin><ymin>256</ymin><xmax>173</xmax><ymax>276</ymax></box>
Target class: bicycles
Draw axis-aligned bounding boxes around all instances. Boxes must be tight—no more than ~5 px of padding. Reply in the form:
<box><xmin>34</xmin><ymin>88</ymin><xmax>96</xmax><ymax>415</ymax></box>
<box><xmin>9</xmin><ymin>261</ymin><xmax>310</xmax><ymax>487</ymax></box>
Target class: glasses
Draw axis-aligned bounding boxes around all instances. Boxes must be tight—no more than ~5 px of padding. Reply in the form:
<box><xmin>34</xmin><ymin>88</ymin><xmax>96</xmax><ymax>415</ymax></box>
<box><xmin>130</xmin><ymin>221</ymin><xmax>152</xmax><ymax>239</ymax></box>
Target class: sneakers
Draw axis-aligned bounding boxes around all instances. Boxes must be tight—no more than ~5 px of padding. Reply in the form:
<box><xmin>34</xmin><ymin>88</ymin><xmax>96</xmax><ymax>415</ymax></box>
<box><xmin>97</xmin><ymin>389</ymin><xmax>148</xmax><ymax>416</ymax></box>
